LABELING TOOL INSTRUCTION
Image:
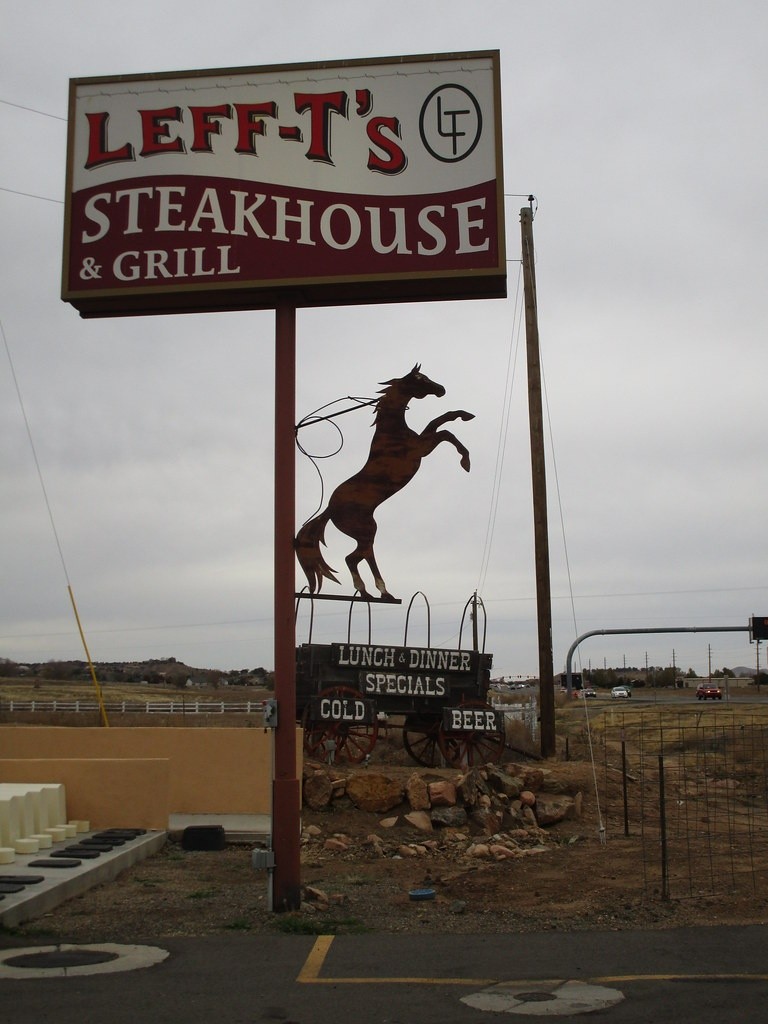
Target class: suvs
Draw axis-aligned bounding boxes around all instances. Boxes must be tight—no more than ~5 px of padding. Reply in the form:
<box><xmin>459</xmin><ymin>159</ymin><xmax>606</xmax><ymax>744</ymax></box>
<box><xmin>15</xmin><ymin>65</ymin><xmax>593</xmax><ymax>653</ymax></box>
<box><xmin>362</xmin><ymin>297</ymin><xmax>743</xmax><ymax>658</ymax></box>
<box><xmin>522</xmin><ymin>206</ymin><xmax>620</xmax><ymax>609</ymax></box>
<box><xmin>695</xmin><ymin>683</ymin><xmax>722</xmax><ymax>700</ymax></box>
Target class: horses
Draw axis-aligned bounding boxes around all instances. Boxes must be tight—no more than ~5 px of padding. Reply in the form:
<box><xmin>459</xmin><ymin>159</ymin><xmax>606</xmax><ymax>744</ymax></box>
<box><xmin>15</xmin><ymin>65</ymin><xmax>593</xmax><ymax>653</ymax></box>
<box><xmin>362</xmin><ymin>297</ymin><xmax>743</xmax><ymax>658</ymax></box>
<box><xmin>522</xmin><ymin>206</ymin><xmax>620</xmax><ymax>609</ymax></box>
<box><xmin>296</xmin><ymin>363</ymin><xmax>476</xmax><ymax>600</ymax></box>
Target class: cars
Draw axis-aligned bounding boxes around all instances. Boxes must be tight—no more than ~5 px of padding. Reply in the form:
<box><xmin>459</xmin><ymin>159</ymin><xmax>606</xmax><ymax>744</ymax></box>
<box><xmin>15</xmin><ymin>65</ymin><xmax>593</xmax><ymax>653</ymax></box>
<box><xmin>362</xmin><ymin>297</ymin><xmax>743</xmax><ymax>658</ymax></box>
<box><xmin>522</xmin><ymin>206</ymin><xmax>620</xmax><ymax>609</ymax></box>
<box><xmin>580</xmin><ymin>689</ymin><xmax>596</xmax><ymax>698</ymax></box>
<box><xmin>611</xmin><ymin>687</ymin><xmax>628</xmax><ymax>699</ymax></box>
<box><xmin>560</xmin><ymin>688</ymin><xmax>566</xmax><ymax>693</ymax></box>
<box><xmin>624</xmin><ymin>685</ymin><xmax>632</xmax><ymax>698</ymax></box>
<box><xmin>510</xmin><ymin>683</ymin><xmax>530</xmax><ymax>690</ymax></box>
